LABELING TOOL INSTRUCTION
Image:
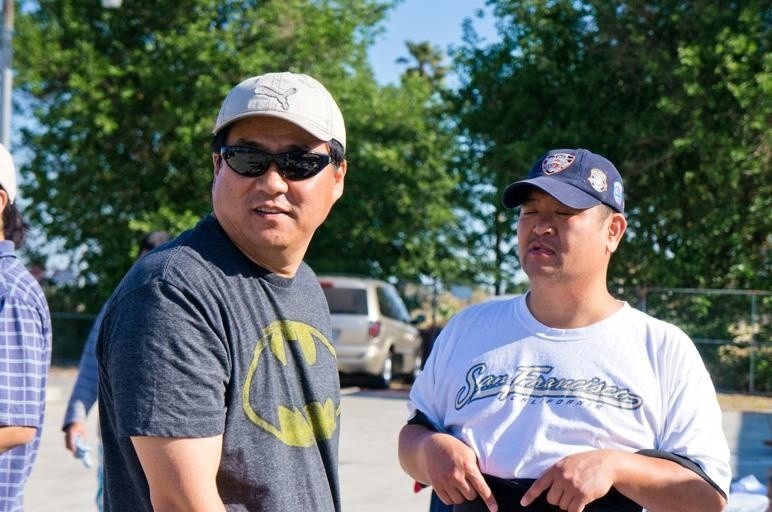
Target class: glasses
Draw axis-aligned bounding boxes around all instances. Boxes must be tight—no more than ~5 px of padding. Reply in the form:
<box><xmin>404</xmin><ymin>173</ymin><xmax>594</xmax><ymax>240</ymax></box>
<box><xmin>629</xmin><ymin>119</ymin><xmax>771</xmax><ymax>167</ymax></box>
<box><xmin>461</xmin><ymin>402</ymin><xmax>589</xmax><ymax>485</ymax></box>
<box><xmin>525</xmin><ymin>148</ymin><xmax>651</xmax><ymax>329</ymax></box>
<box><xmin>219</xmin><ymin>145</ymin><xmax>335</xmax><ymax>181</ymax></box>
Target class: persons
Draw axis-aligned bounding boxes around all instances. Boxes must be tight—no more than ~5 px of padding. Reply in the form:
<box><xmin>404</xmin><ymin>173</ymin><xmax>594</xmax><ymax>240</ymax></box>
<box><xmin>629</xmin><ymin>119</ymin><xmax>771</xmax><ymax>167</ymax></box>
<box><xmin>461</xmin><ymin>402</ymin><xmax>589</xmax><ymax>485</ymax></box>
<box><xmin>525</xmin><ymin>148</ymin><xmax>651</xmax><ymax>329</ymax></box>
<box><xmin>0</xmin><ymin>138</ymin><xmax>53</xmax><ymax>511</ymax></box>
<box><xmin>59</xmin><ymin>227</ymin><xmax>174</xmax><ymax>511</ymax></box>
<box><xmin>395</xmin><ymin>146</ymin><xmax>733</xmax><ymax>512</ymax></box>
<box><xmin>93</xmin><ymin>71</ymin><xmax>348</xmax><ymax>510</ymax></box>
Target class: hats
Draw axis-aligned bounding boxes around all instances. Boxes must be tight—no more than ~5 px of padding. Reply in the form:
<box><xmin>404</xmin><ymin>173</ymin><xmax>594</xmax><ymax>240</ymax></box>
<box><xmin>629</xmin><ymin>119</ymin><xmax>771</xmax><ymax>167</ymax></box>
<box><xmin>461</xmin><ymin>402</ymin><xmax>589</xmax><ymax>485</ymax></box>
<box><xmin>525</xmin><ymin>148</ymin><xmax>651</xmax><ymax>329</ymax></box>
<box><xmin>501</xmin><ymin>149</ymin><xmax>626</xmax><ymax>214</ymax></box>
<box><xmin>213</xmin><ymin>72</ymin><xmax>347</xmax><ymax>155</ymax></box>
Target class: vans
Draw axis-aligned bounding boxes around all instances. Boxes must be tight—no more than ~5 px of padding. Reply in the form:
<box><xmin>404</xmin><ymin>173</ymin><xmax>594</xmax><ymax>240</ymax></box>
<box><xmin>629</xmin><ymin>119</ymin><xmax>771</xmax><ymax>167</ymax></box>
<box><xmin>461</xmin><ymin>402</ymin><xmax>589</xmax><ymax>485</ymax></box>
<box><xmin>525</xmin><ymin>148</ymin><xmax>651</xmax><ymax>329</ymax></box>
<box><xmin>315</xmin><ymin>275</ymin><xmax>426</xmax><ymax>390</ymax></box>
